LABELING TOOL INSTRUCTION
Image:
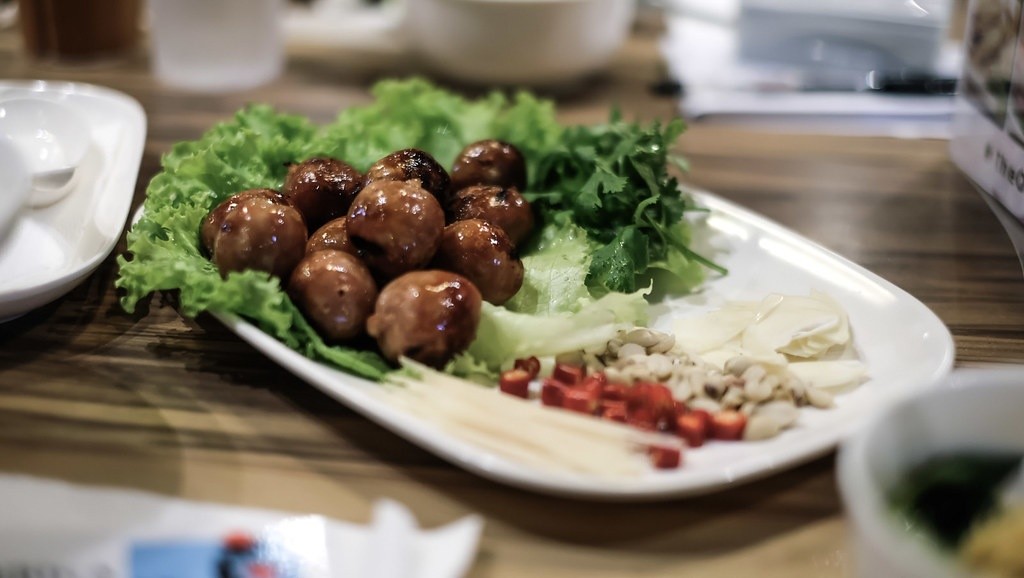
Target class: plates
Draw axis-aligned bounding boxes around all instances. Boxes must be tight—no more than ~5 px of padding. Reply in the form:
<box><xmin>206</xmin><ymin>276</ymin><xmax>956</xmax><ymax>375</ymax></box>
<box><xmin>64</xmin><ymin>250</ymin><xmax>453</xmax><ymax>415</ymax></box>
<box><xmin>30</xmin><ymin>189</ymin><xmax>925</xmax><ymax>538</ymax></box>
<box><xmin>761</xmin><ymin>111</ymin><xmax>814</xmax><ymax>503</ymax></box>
<box><xmin>135</xmin><ymin>151</ymin><xmax>954</xmax><ymax>506</ymax></box>
<box><xmin>0</xmin><ymin>75</ymin><xmax>149</xmax><ymax>325</ymax></box>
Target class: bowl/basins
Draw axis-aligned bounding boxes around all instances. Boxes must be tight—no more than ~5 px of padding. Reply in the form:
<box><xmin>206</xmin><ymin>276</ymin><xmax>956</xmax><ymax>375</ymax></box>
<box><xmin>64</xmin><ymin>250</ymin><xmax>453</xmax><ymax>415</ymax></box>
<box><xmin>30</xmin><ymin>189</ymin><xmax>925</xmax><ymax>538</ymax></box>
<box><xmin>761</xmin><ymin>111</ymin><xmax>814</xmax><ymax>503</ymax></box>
<box><xmin>406</xmin><ymin>1</ymin><xmax>636</xmax><ymax>89</ymax></box>
<box><xmin>830</xmin><ymin>359</ymin><xmax>1023</xmax><ymax>578</ymax></box>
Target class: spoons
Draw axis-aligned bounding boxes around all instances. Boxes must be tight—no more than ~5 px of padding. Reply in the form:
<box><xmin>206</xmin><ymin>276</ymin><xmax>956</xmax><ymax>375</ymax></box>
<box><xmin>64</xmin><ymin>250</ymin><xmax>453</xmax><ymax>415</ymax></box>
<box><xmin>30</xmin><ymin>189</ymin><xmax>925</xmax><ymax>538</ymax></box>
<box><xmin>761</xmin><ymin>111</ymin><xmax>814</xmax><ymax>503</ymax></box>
<box><xmin>2</xmin><ymin>132</ymin><xmax>88</xmax><ymax>238</ymax></box>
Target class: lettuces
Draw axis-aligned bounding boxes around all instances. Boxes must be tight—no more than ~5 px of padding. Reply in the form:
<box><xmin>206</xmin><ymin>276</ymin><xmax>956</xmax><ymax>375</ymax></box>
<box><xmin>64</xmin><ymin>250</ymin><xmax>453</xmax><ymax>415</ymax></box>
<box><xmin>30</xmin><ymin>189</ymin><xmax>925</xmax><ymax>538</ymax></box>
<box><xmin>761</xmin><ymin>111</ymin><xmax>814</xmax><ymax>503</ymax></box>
<box><xmin>115</xmin><ymin>78</ymin><xmax>654</xmax><ymax>392</ymax></box>
